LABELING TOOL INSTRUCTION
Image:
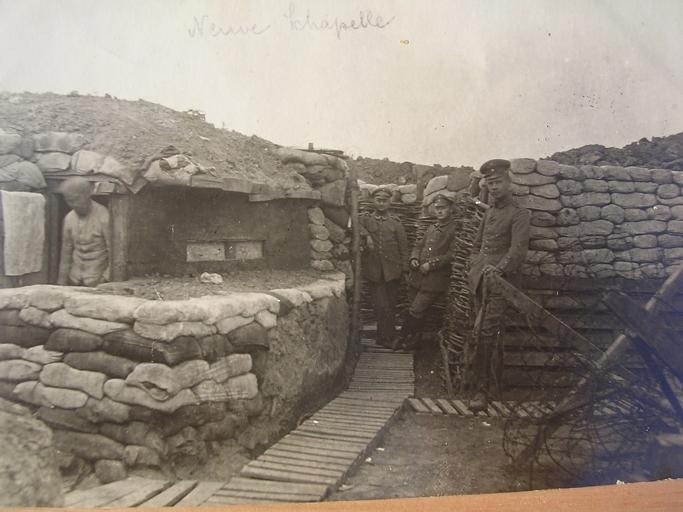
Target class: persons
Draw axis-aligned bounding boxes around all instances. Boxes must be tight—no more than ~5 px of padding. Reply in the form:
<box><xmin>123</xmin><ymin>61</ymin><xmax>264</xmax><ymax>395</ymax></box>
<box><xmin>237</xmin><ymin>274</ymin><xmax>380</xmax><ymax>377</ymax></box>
<box><xmin>468</xmin><ymin>159</ymin><xmax>530</xmax><ymax>411</ymax></box>
<box><xmin>400</xmin><ymin>193</ymin><xmax>455</xmax><ymax>338</ymax></box>
<box><xmin>57</xmin><ymin>177</ymin><xmax>111</xmax><ymax>287</ymax></box>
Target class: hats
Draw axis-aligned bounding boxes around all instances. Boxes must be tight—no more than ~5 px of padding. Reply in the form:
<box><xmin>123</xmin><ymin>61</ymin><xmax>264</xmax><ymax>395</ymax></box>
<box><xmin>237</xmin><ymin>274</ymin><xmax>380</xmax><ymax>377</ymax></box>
<box><xmin>372</xmin><ymin>186</ymin><xmax>394</xmax><ymax>197</ymax></box>
<box><xmin>480</xmin><ymin>159</ymin><xmax>511</xmax><ymax>181</ymax></box>
<box><xmin>430</xmin><ymin>193</ymin><xmax>456</xmax><ymax>208</ymax></box>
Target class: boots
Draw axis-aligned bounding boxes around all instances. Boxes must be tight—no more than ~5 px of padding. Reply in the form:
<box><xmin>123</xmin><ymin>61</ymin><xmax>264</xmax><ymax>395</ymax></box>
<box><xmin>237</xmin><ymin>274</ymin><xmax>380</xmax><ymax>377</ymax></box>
<box><xmin>468</xmin><ymin>334</ymin><xmax>504</xmax><ymax>409</ymax></box>
<box><xmin>376</xmin><ymin>315</ymin><xmax>426</xmax><ymax>351</ymax></box>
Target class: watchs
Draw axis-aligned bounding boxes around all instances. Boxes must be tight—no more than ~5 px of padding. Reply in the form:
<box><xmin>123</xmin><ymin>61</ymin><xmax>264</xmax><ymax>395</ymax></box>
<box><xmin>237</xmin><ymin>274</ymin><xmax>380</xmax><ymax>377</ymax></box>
<box><xmin>358</xmin><ymin>187</ymin><xmax>412</xmax><ymax>350</ymax></box>
